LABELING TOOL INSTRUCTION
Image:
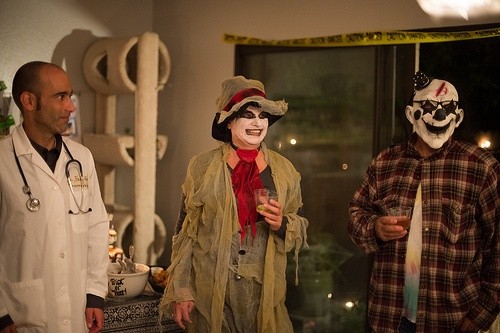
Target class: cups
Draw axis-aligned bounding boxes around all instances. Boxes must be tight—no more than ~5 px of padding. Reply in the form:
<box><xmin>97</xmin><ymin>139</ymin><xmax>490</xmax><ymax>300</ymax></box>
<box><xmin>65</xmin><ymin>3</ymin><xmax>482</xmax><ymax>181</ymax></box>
<box><xmin>386</xmin><ymin>206</ymin><xmax>412</xmax><ymax>230</ymax></box>
<box><xmin>254</xmin><ymin>189</ymin><xmax>278</xmax><ymax>213</ymax></box>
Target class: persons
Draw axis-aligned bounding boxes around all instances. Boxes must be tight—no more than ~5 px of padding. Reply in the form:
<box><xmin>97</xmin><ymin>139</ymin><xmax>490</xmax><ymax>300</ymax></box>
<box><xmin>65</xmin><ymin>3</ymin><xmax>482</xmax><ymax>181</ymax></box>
<box><xmin>0</xmin><ymin>60</ymin><xmax>108</xmax><ymax>332</ymax></box>
<box><xmin>347</xmin><ymin>71</ymin><xmax>500</xmax><ymax>333</ymax></box>
<box><xmin>168</xmin><ymin>75</ymin><xmax>307</xmax><ymax>333</ymax></box>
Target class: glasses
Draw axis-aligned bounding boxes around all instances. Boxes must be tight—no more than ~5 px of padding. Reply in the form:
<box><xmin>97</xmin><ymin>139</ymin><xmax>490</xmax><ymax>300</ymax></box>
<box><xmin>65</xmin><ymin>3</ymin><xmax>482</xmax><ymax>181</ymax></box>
<box><xmin>412</xmin><ymin>100</ymin><xmax>460</xmax><ymax>110</ymax></box>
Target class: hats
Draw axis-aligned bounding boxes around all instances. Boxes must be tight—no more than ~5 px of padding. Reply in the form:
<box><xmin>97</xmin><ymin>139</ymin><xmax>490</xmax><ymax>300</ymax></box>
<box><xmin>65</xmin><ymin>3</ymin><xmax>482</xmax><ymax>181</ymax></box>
<box><xmin>212</xmin><ymin>75</ymin><xmax>289</xmax><ymax>143</ymax></box>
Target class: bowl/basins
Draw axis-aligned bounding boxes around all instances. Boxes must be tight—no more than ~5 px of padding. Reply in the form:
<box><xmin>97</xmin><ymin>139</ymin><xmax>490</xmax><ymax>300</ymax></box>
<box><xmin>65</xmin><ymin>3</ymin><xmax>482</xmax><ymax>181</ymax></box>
<box><xmin>106</xmin><ymin>263</ymin><xmax>150</xmax><ymax>300</ymax></box>
